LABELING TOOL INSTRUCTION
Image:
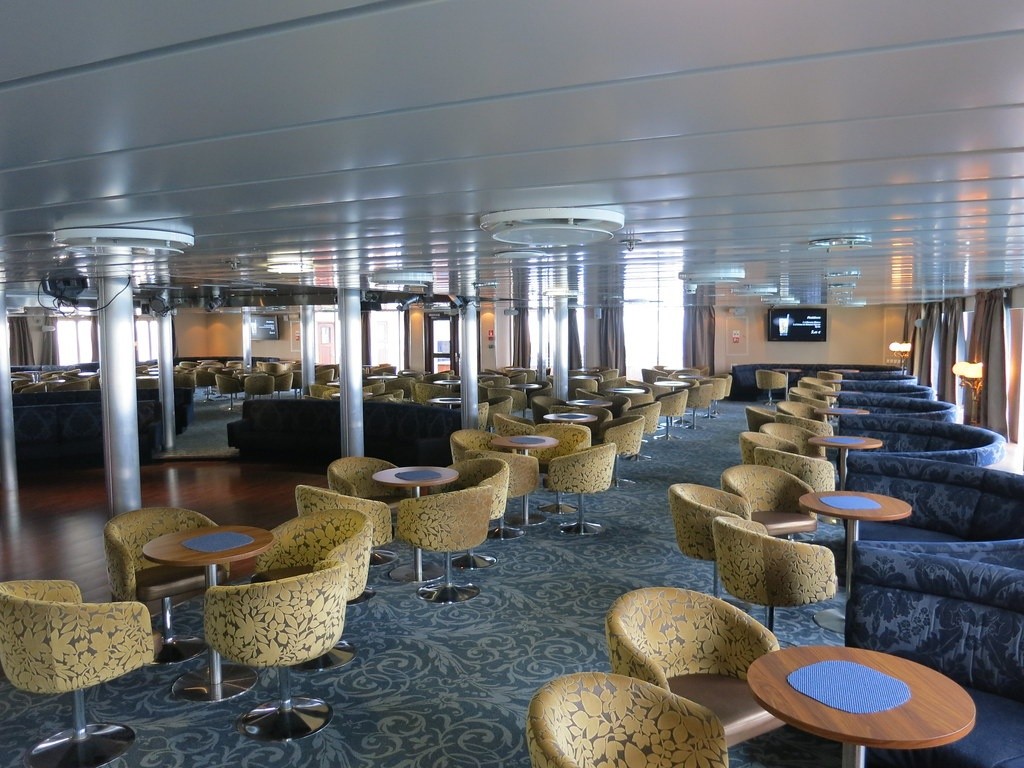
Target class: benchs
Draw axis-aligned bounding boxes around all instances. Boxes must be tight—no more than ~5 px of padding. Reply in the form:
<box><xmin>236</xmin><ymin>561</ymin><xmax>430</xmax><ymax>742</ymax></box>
<box><xmin>731</xmin><ymin>364</ymin><xmax>1024</xmax><ymax>768</ymax></box>
<box><xmin>227</xmin><ymin>400</ymin><xmax>460</xmax><ymax>467</ymax></box>
<box><xmin>12</xmin><ymin>387</ymin><xmax>193</xmax><ymax>435</ymax></box>
<box><xmin>13</xmin><ymin>400</ymin><xmax>163</xmax><ymax>470</ymax></box>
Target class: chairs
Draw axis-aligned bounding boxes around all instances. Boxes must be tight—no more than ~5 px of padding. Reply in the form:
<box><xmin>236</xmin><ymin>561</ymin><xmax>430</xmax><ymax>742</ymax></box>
<box><xmin>0</xmin><ymin>361</ymin><xmax>842</xmax><ymax>768</ymax></box>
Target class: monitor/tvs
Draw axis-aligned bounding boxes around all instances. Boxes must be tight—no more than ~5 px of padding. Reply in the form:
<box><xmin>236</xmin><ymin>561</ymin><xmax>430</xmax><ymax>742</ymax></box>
<box><xmin>768</xmin><ymin>307</ymin><xmax>827</xmax><ymax>342</ymax></box>
<box><xmin>249</xmin><ymin>315</ymin><xmax>278</xmax><ymax>341</ymax></box>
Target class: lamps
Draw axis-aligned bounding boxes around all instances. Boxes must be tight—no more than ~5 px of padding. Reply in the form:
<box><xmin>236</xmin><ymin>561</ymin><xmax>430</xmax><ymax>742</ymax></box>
<box><xmin>54</xmin><ymin>228</ymin><xmax>195</xmax><ymax>254</ymax></box>
<box><xmin>889</xmin><ymin>341</ymin><xmax>911</xmax><ymax>374</ymax></box>
<box><xmin>952</xmin><ymin>362</ymin><xmax>983</xmax><ymax>425</ymax></box>
<box><xmin>731</xmin><ymin>287</ymin><xmax>777</xmax><ymax>296</ymax></box>
<box><xmin>808</xmin><ymin>237</ymin><xmax>872</xmax><ymax>253</ymax></box>
<box><xmin>369</xmin><ymin>273</ymin><xmax>434</xmax><ymax>287</ymax></box>
<box><xmin>479</xmin><ymin>208</ymin><xmax>642</xmax><ymax>252</ymax></box>
<box><xmin>679</xmin><ymin>273</ymin><xmax>745</xmax><ymax>285</ymax></box>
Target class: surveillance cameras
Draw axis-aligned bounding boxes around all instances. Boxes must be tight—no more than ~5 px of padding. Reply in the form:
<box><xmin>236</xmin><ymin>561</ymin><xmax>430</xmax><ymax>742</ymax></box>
<box><xmin>685</xmin><ymin>284</ymin><xmax>697</xmax><ymax>294</ymax></box>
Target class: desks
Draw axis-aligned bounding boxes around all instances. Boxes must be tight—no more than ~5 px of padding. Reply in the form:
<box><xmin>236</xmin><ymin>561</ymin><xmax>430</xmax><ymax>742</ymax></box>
<box><xmin>606</xmin><ymin>388</ymin><xmax>645</xmax><ymax>394</ymax></box>
<box><xmin>425</xmin><ymin>397</ymin><xmax>461</xmax><ymax>410</ymax></box>
<box><xmin>505</xmin><ymin>384</ymin><xmax>542</xmax><ymax>418</ymax></box>
<box><xmin>371</xmin><ymin>467</ymin><xmax>460</xmax><ymax>583</ymax></box>
<box><xmin>829</xmin><ymin>369</ymin><xmax>859</xmax><ymax>373</ymax></box>
<box><xmin>569</xmin><ymin>368</ymin><xmax>599</xmax><ymax>373</ymax></box>
<box><xmin>332</xmin><ymin>392</ymin><xmax>373</xmax><ymax>399</ymax></box>
<box><xmin>678</xmin><ymin>375</ymin><xmax>710</xmax><ymax>380</ymax></box>
<box><xmin>491</xmin><ymin>435</ymin><xmax>560</xmax><ymax>526</ymax></box>
<box><xmin>808</xmin><ymin>436</ymin><xmax>883</xmax><ymax>490</ymax></box>
<box><xmin>812</xmin><ymin>408</ymin><xmax>870</xmax><ymax>415</ymax></box>
<box><xmin>478</xmin><ymin>375</ymin><xmax>504</xmax><ymax>378</ymax></box>
<box><xmin>432</xmin><ymin>380</ymin><xmax>460</xmax><ymax>390</ymax></box>
<box><xmin>745</xmin><ymin>645</ymin><xmax>976</xmax><ymax>768</ymax></box>
<box><xmin>823</xmin><ymin>380</ymin><xmax>842</xmax><ymax>383</ymax></box>
<box><xmin>142</xmin><ymin>526</ymin><xmax>273</xmax><ymax>703</ymax></box>
<box><xmin>367</xmin><ymin>376</ymin><xmax>398</xmax><ymax>383</ymax></box>
<box><xmin>543</xmin><ymin>413</ymin><xmax>599</xmax><ymax>424</ymax></box>
<box><xmin>28</xmin><ymin>380</ymin><xmax>66</xmax><ymax>392</ymax></box>
<box><xmin>568</xmin><ymin>375</ymin><xmax>601</xmax><ymax>380</ymax></box>
<box><xmin>652</xmin><ymin>381</ymin><xmax>690</xmax><ymax>426</ymax></box>
<box><xmin>773</xmin><ymin>369</ymin><xmax>802</xmax><ymax>402</ymax></box>
<box><xmin>327</xmin><ymin>382</ymin><xmax>340</xmax><ymax>388</ymax></box>
<box><xmin>800</xmin><ymin>490</ymin><xmax>912</xmax><ymax>633</ymax></box>
<box><xmin>566</xmin><ymin>400</ymin><xmax>613</xmax><ymax>406</ymax></box>
<box><xmin>403</xmin><ymin>371</ymin><xmax>431</xmax><ymax>376</ymax></box>
<box><xmin>824</xmin><ymin>391</ymin><xmax>863</xmax><ymax>396</ymax></box>
<box><xmin>507</xmin><ymin>367</ymin><xmax>538</xmax><ymax>371</ymax></box>
